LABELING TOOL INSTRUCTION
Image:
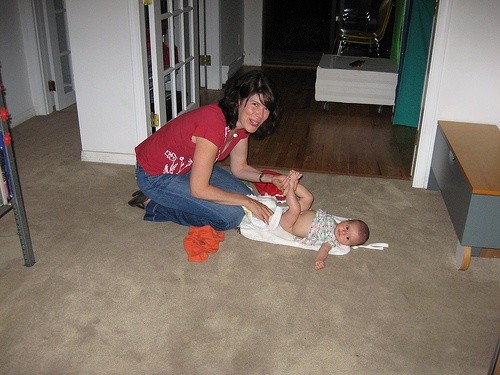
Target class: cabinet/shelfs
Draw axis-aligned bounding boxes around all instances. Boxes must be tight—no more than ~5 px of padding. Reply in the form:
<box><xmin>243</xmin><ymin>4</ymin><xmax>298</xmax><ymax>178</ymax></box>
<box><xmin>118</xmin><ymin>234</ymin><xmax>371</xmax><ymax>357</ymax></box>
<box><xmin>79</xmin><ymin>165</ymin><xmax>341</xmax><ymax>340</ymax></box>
<box><xmin>427</xmin><ymin>120</ymin><xmax>500</xmax><ymax>271</ymax></box>
<box><xmin>314</xmin><ymin>53</ymin><xmax>398</xmax><ymax>115</ymax></box>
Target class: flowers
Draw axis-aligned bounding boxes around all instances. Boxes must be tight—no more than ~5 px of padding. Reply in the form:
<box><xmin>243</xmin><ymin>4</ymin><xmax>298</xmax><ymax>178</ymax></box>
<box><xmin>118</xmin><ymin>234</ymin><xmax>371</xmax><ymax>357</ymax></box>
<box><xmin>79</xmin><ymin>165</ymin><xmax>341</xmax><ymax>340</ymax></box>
<box><xmin>2</xmin><ymin>131</ymin><xmax>12</xmax><ymax>147</ymax></box>
<box><xmin>0</xmin><ymin>107</ymin><xmax>10</xmax><ymax>121</ymax></box>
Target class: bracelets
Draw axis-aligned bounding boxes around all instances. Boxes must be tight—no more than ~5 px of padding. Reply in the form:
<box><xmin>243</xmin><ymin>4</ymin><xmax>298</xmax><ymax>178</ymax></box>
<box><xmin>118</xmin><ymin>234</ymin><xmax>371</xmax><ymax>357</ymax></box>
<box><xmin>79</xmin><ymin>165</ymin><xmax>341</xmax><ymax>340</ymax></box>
<box><xmin>259</xmin><ymin>173</ymin><xmax>266</xmax><ymax>182</ymax></box>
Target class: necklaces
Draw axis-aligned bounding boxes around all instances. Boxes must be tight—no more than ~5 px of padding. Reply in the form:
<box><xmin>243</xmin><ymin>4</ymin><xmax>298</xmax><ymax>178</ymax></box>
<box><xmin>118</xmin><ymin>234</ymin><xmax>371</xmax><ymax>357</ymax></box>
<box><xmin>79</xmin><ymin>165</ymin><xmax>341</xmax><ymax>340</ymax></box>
<box><xmin>232</xmin><ymin>130</ymin><xmax>238</xmax><ymax>138</ymax></box>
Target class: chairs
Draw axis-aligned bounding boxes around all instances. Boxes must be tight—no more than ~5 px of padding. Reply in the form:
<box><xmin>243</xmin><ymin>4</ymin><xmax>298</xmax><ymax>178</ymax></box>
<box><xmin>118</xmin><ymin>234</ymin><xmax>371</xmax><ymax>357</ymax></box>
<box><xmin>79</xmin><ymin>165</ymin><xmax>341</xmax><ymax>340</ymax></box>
<box><xmin>331</xmin><ymin>0</ymin><xmax>395</xmax><ymax>57</ymax></box>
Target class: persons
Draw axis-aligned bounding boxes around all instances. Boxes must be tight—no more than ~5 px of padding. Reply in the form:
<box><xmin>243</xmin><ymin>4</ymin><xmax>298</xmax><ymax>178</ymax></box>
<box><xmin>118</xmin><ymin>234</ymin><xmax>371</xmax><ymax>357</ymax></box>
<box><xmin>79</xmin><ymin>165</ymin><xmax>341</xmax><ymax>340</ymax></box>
<box><xmin>279</xmin><ymin>170</ymin><xmax>370</xmax><ymax>270</ymax></box>
<box><xmin>128</xmin><ymin>69</ymin><xmax>281</xmax><ymax>231</ymax></box>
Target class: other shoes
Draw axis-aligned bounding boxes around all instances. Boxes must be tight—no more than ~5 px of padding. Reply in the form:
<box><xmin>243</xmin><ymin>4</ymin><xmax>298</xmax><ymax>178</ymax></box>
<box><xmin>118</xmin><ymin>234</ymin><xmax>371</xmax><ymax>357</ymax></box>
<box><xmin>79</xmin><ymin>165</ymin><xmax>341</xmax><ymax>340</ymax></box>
<box><xmin>128</xmin><ymin>189</ymin><xmax>148</xmax><ymax>209</ymax></box>
